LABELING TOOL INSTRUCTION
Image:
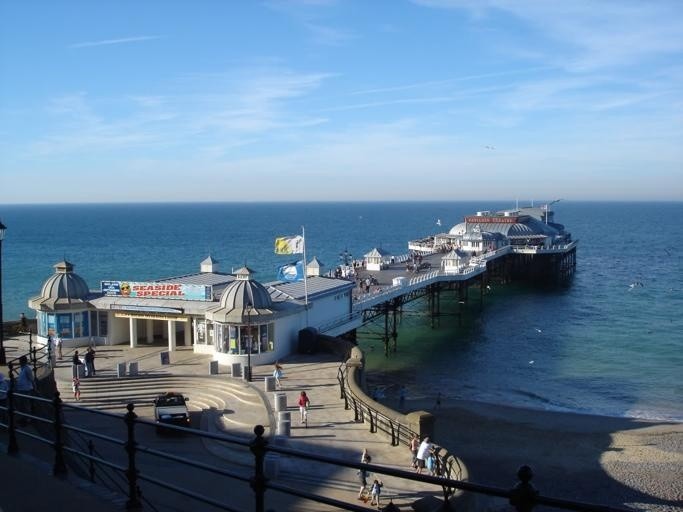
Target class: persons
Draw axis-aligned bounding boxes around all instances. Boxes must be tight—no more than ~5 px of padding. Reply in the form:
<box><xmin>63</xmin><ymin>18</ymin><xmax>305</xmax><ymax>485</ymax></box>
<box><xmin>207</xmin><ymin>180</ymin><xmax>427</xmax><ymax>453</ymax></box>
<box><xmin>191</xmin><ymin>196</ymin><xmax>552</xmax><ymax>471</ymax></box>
<box><xmin>335</xmin><ymin>259</ymin><xmax>378</xmax><ymax>294</ymax></box>
<box><xmin>368</xmin><ymin>478</ymin><xmax>383</xmax><ymax>510</ymax></box>
<box><xmin>410</xmin><ymin>433</ymin><xmax>440</xmax><ymax>477</ymax></box>
<box><xmin>433</xmin><ymin>391</ymin><xmax>442</xmax><ymax>411</ymax></box>
<box><xmin>356</xmin><ymin>455</ymin><xmax>371</xmax><ymax>500</ymax></box>
<box><xmin>72</xmin><ymin>350</ymin><xmax>87</xmax><ymax>372</ymax></box>
<box><xmin>55</xmin><ymin>333</ymin><xmax>62</xmax><ymax>360</ymax></box>
<box><xmin>271</xmin><ymin>363</ymin><xmax>283</xmax><ymax>386</ymax></box>
<box><xmin>295</xmin><ymin>391</ymin><xmax>310</xmax><ymax>422</ymax></box>
<box><xmin>398</xmin><ymin>385</ymin><xmax>408</xmax><ymax>409</ymax></box>
<box><xmin>18</xmin><ymin>312</ymin><xmax>26</xmax><ymax>331</ymax></box>
<box><xmin>70</xmin><ymin>376</ymin><xmax>81</xmax><ymax>400</ymax></box>
<box><xmin>14</xmin><ymin>355</ymin><xmax>36</xmax><ymax>425</ymax></box>
<box><xmin>87</xmin><ymin>345</ymin><xmax>95</xmax><ymax>375</ymax></box>
<box><xmin>0</xmin><ymin>371</ymin><xmax>10</xmax><ymax>430</ymax></box>
<box><xmin>358</xmin><ymin>448</ymin><xmax>368</xmax><ymax>464</ymax></box>
<box><xmin>83</xmin><ymin>348</ymin><xmax>93</xmax><ymax>377</ymax></box>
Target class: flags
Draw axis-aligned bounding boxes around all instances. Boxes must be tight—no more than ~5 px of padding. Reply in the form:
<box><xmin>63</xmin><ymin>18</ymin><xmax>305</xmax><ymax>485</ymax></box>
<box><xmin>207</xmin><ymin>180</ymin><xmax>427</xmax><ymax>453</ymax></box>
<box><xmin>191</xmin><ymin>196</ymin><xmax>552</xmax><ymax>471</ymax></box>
<box><xmin>275</xmin><ymin>258</ymin><xmax>304</xmax><ymax>282</ymax></box>
<box><xmin>272</xmin><ymin>234</ymin><xmax>304</xmax><ymax>255</ymax></box>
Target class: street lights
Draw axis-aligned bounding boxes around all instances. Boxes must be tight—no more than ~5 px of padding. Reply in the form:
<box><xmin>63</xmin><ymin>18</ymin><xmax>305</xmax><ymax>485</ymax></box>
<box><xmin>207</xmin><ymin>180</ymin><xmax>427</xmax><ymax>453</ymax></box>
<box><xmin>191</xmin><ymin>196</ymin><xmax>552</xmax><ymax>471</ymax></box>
<box><xmin>0</xmin><ymin>222</ymin><xmax>7</xmax><ymax>366</ymax></box>
<box><xmin>245</xmin><ymin>301</ymin><xmax>253</xmax><ymax>383</ymax></box>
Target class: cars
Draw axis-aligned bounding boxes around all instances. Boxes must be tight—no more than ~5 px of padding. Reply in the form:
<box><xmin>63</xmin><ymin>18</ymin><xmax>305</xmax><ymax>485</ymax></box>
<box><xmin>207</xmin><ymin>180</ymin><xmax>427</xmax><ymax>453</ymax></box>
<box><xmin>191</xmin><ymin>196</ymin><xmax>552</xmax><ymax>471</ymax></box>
<box><xmin>152</xmin><ymin>393</ymin><xmax>192</xmax><ymax>428</ymax></box>
